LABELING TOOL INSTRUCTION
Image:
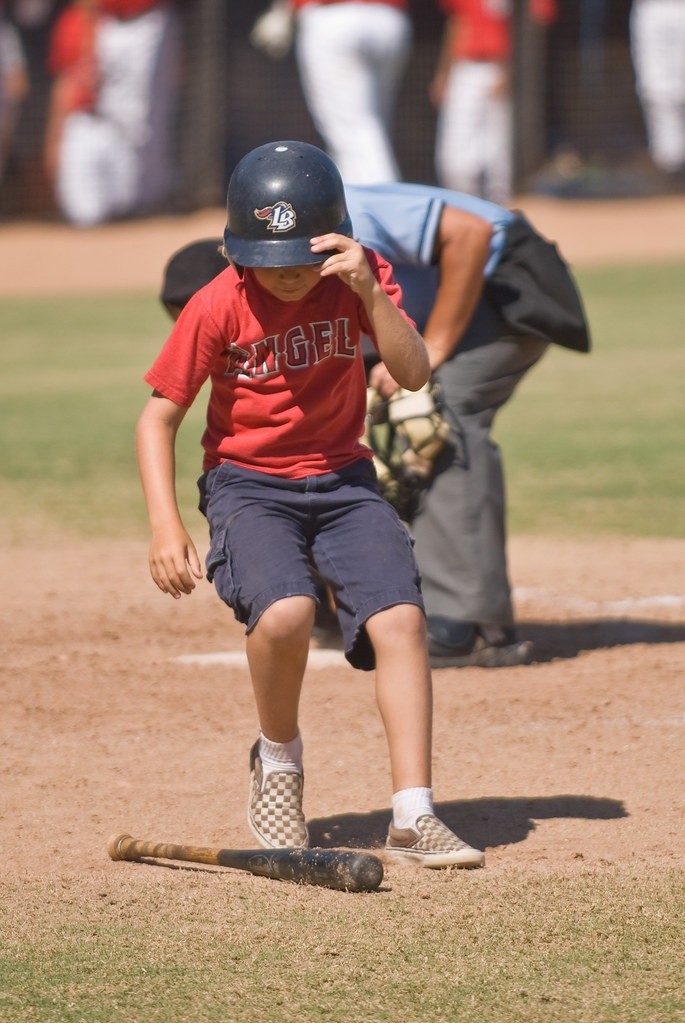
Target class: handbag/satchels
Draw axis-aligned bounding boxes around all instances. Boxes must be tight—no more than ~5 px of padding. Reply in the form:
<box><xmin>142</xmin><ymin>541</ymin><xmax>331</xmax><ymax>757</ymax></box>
<box><xmin>484</xmin><ymin>209</ymin><xmax>592</xmax><ymax>352</ymax></box>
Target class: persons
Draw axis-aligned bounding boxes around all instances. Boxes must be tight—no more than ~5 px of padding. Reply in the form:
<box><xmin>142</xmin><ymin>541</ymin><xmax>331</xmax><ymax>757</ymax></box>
<box><xmin>0</xmin><ymin>0</ymin><xmax>167</xmax><ymax>223</ymax></box>
<box><xmin>631</xmin><ymin>0</ymin><xmax>685</xmax><ymax>192</ymax></box>
<box><xmin>159</xmin><ymin>182</ymin><xmax>592</xmax><ymax>666</ymax></box>
<box><xmin>134</xmin><ymin>140</ymin><xmax>484</xmax><ymax>868</ymax></box>
<box><xmin>431</xmin><ymin>0</ymin><xmax>553</xmax><ymax>204</ymax></box>
<box><xmin>254</xmin><ymin>0</ymin><xmax>414</xmax><ymax>182</ymax></box>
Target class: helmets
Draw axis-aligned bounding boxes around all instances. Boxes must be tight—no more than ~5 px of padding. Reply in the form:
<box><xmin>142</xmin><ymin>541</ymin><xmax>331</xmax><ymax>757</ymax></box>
<box><xmin>224</xmin><ymin>140</ymin><xmax>353</xmax><ymax>269</ymax></box>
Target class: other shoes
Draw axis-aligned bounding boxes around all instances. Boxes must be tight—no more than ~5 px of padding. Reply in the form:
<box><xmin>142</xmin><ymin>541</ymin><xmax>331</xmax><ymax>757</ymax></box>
<box><xmin>426</xmin><ymin>616</ymin><xmax>534</xmax><ymax>665</ymax></box>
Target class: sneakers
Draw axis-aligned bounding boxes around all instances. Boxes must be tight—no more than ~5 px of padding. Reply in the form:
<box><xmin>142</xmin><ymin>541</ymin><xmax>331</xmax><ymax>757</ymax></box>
<box><xmin>384</xmin><ymin>813</ymin><xmax>486</xmax><ymax>869</ymax></box>
<box><xmin>247</xmin><ymin>739</ymin><xmax>311</xmax><ymax>850</ymax></box>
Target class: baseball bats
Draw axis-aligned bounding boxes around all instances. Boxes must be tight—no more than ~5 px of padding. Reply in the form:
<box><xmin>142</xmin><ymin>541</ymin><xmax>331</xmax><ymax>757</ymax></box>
<box><xmin>108</xmin><ymin>833</ymin><xmax>385</xmax><ymax>892</ymax></box>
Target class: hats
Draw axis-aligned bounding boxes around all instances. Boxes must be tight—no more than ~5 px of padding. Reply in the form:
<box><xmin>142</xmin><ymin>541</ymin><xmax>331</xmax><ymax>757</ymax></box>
<box><xmin>160</xmin><ymin>239</ymin><xmax>228</xmax><ymax>323</ymax></box>
